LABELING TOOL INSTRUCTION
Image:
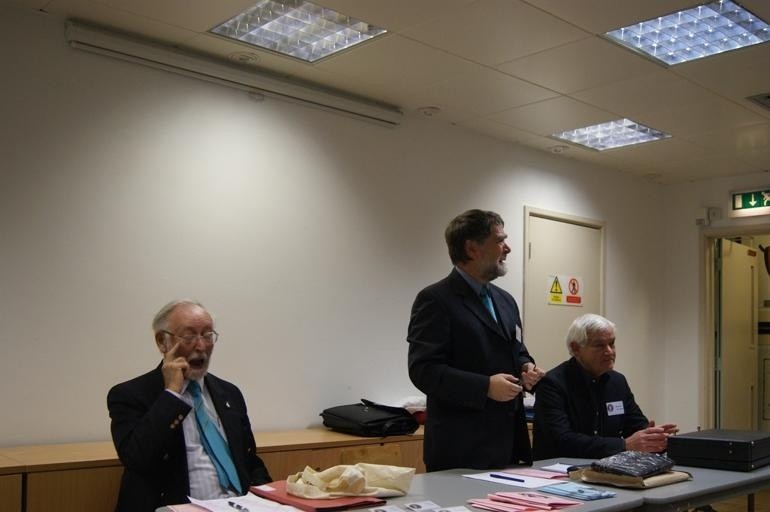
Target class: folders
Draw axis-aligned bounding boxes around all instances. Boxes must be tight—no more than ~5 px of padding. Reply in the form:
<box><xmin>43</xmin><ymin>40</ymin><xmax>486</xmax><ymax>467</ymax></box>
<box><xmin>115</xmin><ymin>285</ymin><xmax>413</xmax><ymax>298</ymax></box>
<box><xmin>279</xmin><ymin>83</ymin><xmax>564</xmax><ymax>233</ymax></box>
<box><xmin>249</xmin><ymin>479</ymin><xmax>387</xmax><ymax>512</ymax></box>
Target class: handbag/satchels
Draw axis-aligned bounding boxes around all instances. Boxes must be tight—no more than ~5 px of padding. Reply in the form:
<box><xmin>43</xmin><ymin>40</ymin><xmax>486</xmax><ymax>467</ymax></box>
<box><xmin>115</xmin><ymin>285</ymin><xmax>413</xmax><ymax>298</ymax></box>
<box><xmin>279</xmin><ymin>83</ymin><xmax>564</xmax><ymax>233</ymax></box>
<box><xmin>567</xmin><ymin>451</ymin><xmax>692</xmax><ymax>490</ymax></box>
<box><xmin>319</xmin><ymin>399</ymin><xmax>419</xmax><ymax>438</ymax></box>
<box><xmin>591</xmin><ymin>451</ymin><xmax>674</xmax><ymax>480</ymax></box>
<box><xmin>286</xmin><ymin>463</ymin><xmax>416</xmax><ymax>499</ymax></box>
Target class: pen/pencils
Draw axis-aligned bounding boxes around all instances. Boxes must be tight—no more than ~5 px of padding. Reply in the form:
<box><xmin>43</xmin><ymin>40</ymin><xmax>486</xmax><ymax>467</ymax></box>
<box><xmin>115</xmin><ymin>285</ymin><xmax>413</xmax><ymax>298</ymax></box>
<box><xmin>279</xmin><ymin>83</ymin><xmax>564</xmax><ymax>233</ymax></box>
<box><xmin>228</xmin><ymin>500</ymin><xmax>249</xmax><ymax>512</ymax></box>
<box><xmin>490</xmin><ymin>474</ymin><xmax>524</xmax><ymax>484</ymax></box>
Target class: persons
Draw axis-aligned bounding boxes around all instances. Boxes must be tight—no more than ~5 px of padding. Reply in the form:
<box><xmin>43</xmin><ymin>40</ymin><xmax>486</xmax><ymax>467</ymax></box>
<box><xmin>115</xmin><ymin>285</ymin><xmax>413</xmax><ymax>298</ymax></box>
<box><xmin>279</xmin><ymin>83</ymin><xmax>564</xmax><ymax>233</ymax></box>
<box><xmin>533</xmin><ymin>312</ymin><xmax>678</xmax><ymax>459</ymax></box>
<box><xmin>106</xmin><ymin>297</ymin><xmax>275</xmax><ymax>508</ymax></box>
<box><xmin>406</xmin><ymin>208</ymin><xmax>547</xmax><ymax>473</ymax></box>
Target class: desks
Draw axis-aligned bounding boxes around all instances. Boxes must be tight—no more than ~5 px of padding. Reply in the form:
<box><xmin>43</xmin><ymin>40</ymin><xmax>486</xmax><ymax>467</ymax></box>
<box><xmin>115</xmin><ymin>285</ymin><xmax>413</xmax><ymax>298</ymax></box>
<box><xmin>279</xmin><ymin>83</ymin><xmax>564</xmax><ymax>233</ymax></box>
<box><xmin>445</xmin><ymin>458</ymin><xmax>770</xmax><ymax>512</ymax></box>
<box><xmin>156</xmin><ymin>469</ymin><xmax>644</xmax><ymax>511</ymax></box>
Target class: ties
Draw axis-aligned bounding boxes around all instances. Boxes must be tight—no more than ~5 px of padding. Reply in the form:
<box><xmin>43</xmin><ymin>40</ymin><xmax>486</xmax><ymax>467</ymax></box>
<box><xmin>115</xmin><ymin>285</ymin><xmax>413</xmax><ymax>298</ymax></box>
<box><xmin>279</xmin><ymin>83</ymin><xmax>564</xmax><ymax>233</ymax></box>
<box><xmin>186</xmin><ymin>380</ymin><xmax>242</xmax><ymax>496</ymax></box>
<box><xmin>481</xmin><ymin>285</ymin><xmax>498</xmax><ymax>326</ymax></box>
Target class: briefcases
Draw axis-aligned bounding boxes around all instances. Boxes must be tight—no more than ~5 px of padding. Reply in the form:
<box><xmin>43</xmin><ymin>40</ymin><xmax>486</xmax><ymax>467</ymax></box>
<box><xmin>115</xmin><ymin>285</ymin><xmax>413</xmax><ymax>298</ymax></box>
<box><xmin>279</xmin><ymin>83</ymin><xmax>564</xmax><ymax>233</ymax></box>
<box><xmin>668</xmin><ymin>429</ymin><xmax>770</xmax><ymax>472</ymax></box>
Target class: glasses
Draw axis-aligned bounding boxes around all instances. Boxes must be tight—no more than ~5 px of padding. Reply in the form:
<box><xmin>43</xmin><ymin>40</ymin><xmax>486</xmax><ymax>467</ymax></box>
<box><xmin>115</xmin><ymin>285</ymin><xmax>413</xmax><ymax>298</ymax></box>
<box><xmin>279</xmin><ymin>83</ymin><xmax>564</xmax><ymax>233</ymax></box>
<box><xmin>159</xmin><ymin>330</ymin><xmax>219</xmax><ymax>346</ymax></box>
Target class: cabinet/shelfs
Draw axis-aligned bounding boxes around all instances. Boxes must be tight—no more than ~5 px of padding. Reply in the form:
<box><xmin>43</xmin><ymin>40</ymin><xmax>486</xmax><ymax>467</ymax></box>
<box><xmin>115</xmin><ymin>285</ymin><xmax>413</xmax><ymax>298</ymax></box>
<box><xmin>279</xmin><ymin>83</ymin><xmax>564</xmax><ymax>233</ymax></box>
<box><xmin>0</xmin><ymin>422</ymin><xmax>533</xmax><ymax>508</ymax></box>
